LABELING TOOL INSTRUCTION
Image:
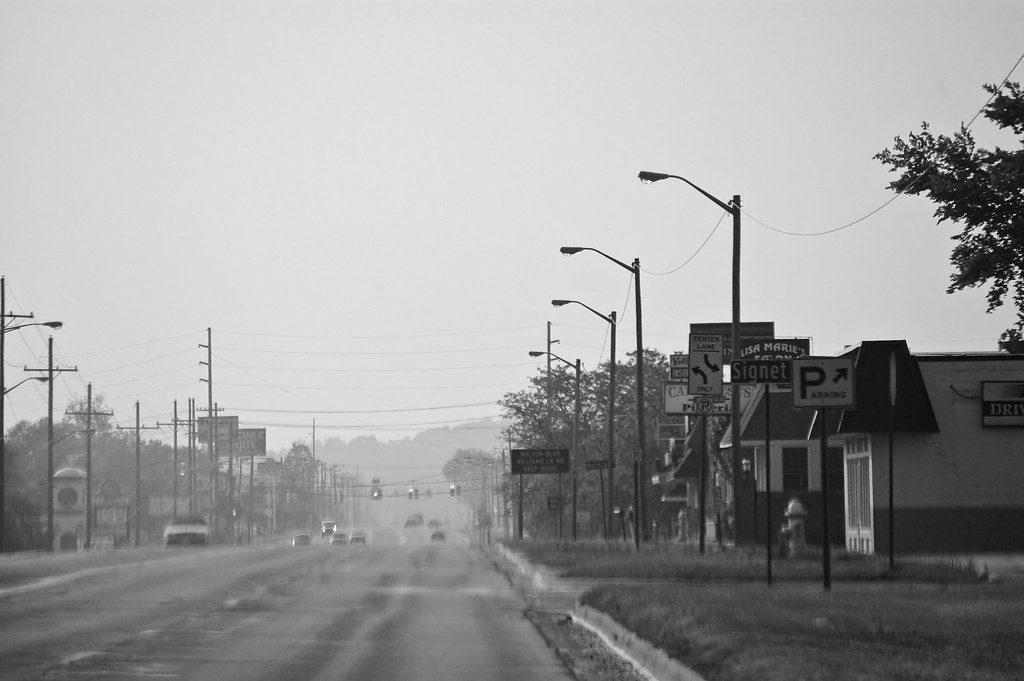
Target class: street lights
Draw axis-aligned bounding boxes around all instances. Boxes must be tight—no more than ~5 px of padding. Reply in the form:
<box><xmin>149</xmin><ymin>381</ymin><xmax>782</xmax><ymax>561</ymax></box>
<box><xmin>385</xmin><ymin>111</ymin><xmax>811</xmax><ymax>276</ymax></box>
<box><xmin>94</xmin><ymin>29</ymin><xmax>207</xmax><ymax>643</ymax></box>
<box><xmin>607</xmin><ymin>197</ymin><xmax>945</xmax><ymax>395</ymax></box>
<box><xmin>0</xmin><ymin>278</ymin><xmax>64</xmax><ymax>545</ymax></box>
<box><xmin>551</xmin><ymin>298</ymin><xmax>615</xmax><ymax>540</ymax></box>
<box><xmin>528</xmin><ymin>352</ymin><xmax>583</xmax><ymax>542</ymax></box>
<box><xmin>45</xmin><ymin>427</ymin><xmax>96</xmax><ymax>550</ymax></box>
<box><xmin>559</xmin><ymin>244</ymin><xmax>649</xmax><ymax>539</ymax></box>
<box><xmin>637</xmin><ymin>168</ymin><xmax>749</xmax><ymax>548</ymax></box>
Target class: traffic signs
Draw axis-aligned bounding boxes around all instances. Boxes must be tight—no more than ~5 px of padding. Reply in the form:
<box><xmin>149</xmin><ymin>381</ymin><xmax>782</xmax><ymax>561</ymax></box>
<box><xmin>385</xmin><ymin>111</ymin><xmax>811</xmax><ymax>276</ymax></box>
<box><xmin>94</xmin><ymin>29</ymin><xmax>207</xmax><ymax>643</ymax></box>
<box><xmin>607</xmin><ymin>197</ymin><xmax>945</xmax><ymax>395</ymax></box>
<box><xmin>792</xmin><ymin>356</ymin><xmax>857</xmax><ymax>409</ymax></box>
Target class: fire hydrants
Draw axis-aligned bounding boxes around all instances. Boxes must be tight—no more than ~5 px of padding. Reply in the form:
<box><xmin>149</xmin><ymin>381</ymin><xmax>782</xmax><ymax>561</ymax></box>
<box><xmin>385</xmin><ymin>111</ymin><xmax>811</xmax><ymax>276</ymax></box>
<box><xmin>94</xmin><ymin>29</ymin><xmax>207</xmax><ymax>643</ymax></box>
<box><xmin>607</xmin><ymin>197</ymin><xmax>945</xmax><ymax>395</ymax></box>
<box><xmin>779</xmin><ymin>498</ymin><xmax>808</xmax><ymax>558</ymax></box>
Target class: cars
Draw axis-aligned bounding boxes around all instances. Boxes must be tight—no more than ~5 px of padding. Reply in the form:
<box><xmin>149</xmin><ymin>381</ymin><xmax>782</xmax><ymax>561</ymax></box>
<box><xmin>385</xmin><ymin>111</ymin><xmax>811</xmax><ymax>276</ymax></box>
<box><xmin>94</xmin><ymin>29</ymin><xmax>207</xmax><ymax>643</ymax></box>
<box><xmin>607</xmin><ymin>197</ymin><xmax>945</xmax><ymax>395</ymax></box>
<box><xmin>430</xmin><ymin>530</ymin><xmax>446</xmax><ymax>543</ymax></box>
<box><xmin>348</xmin><ymin>530</ymin><xmax>367</xmax><ymax>545</ymax></box>
<box><xmin>406</xmin><ymin>513</ymin><xmax>441</xmax><ymax>528</ymax></box>
<box><xmin>291</xmin><ymin>533</ymin><xmax>310</xmax><ymax>547</ymax></box>
<box><xmin>329</xmin><ymin>532</ymin><xmax>347</xmax><ymax>546</ymax></box>
<box><xmin>162</xmin><ymin>515</ymin><xmax>211</xmax><ymax>544</ymax></box>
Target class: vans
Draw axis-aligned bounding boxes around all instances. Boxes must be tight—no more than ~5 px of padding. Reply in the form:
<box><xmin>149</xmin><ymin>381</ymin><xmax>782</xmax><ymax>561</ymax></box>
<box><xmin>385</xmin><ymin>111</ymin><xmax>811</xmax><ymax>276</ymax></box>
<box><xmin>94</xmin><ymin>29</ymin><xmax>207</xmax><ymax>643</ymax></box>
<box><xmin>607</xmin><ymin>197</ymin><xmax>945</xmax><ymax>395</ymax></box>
<box><xmin>321</xmin><ymin>521</ymin><xmax>338</xmax><ymax>538</ymax></box>
